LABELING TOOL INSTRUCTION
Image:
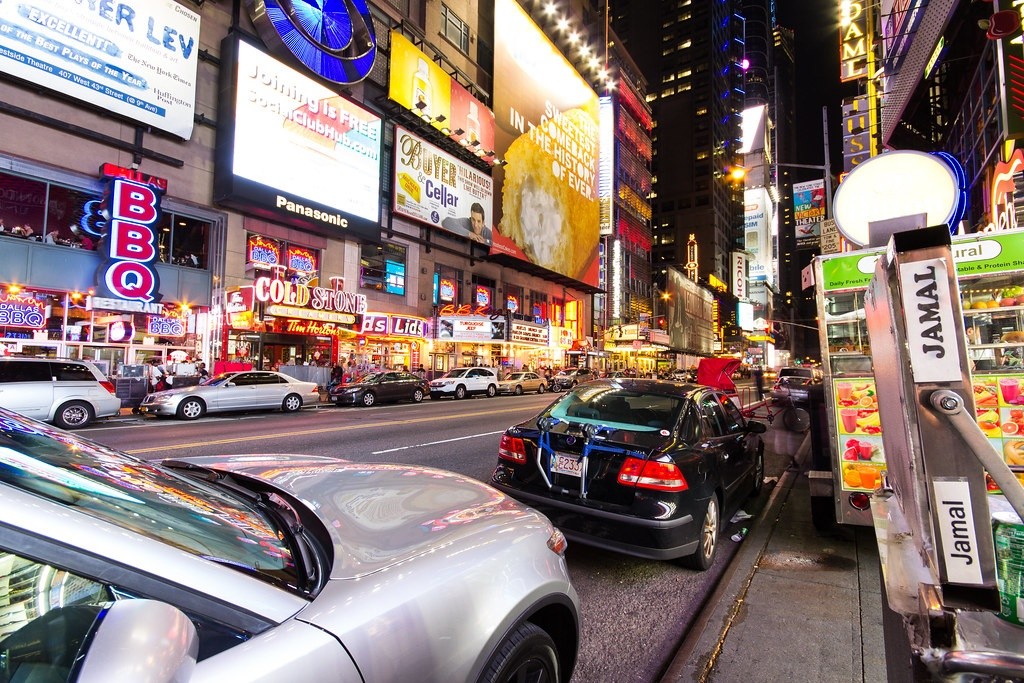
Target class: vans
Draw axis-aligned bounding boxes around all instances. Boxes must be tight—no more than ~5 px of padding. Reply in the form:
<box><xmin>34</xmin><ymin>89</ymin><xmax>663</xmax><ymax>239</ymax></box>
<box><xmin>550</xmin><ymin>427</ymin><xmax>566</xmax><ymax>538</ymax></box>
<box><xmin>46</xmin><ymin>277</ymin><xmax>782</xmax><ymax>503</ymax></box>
<box><xmin>0</xmin><ymin>355</ymin><xmax>123</xmax><ymax>428</ymax></box>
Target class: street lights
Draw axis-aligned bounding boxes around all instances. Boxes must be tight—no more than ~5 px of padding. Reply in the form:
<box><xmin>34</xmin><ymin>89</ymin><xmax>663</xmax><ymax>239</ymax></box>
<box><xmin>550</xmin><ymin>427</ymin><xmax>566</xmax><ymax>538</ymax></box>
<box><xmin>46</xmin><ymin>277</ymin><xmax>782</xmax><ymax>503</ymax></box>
<box><xmin>731</xmin><ymin>163</ymin><xmax>832</xmax><ymax>222</ymax></box>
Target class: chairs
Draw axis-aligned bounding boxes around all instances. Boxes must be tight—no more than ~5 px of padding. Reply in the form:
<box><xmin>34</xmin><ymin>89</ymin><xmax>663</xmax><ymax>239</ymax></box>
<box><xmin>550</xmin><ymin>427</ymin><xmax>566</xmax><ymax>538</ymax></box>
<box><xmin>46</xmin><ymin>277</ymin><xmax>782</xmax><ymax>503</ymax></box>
<box><xmin>574</xmin><ymin>408</ymin><xmax>601</xmax><ymax>420</ymax></box>
<box><xmin>608</xmin><ymin>400</ymin><xmax>639</xmax><ymax>425</ymax></box>
<box><xmin>648</xmin><ymin>411</ymin><xmax>671</xmax><ymax>428</ymax></box>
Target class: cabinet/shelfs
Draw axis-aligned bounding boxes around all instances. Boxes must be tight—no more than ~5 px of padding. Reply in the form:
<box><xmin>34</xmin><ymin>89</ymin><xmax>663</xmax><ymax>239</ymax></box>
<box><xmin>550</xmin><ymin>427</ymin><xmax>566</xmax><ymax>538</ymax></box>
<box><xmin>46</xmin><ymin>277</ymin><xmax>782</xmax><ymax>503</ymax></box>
<box><xmin>809</xmin><ymin>226</ymin><xmax>1024</xmax><ymax>527</ymax></box>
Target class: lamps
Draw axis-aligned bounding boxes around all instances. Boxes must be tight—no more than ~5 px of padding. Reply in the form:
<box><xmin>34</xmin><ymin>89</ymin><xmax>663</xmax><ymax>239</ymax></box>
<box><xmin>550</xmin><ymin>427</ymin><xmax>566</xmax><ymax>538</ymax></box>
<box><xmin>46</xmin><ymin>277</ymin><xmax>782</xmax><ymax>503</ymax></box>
<box><xmin>409</xmin><ymin>99</ymin><xmax>508</xmax><ymax>168</ymax></box>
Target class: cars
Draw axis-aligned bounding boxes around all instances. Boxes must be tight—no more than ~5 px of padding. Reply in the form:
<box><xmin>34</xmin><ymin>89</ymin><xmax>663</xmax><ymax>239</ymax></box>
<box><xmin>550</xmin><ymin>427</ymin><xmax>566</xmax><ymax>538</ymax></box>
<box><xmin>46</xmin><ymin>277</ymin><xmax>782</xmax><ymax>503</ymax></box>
<box><xmin>674</xmin><ymin>369</ymin><xmax>698</xmax><ymax>383</ymax></box>
<box><xmin>490</xmin><ymin>377</ymin><xmax>764</xmax><ymax>571</ymax></box>
<box><xmin>328</xmin><ymin>371</ymin><xmax>431</xmax><ymax>407</ymax></box>
<box><xmin>773</xmin><ymin>367</ymin><xmax>817</xmax><ymax>401</ymax></box>
<box><xmin>603</xmin><ymin>372</ymin><xmax>625</xmax><ymax>377</ymax></box>
<box><xmin>497</xmin><ymin>372</ymin><xmax>550</xmax><ymax>396</ymax></box>
<box><xmin>551</xmin><ymin>367</ymin><xmax>593</xmax><ymax>388</ymax></box>
<box><xmin>0</xmin><ymin>409</ymin><xmax>582</xmax><ymax>683</ymax></box>
<box><xmin>140</xmin><ymin>371</ymin><xmax>320</xmax><ymax>419</ymax></box>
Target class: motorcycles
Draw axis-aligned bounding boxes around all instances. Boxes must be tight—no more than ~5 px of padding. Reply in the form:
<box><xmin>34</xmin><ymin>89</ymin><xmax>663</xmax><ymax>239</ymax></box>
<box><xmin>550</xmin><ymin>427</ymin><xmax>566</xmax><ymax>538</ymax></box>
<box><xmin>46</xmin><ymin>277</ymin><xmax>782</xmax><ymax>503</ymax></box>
<box><xmin>548</xmin><ymin>379</ymin><xmax>561</xmax><ymax>392</ymax></box>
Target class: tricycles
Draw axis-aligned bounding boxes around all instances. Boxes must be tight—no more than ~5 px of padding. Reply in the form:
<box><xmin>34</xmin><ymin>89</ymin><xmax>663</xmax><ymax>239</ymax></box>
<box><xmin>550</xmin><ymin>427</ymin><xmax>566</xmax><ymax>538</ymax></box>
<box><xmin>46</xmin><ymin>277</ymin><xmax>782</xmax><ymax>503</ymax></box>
<box><xmin>697</xmin><ymin>357</ymin><xmax>811</xmax><ymax>433</ymax></box>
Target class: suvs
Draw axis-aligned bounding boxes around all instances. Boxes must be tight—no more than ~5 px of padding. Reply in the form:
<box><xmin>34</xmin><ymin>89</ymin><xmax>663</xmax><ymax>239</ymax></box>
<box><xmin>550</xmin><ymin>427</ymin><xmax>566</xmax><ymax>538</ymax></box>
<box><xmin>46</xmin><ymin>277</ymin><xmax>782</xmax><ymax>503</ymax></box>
<box><xmin>429</xmin><ymin>366</ymin><xmax>497</xmax><ymax>399</ymax></box>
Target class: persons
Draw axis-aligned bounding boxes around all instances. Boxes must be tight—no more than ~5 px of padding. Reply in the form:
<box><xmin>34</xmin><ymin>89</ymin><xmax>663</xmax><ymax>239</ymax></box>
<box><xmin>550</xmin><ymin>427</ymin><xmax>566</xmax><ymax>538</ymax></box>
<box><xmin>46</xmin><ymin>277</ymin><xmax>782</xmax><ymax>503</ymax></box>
<box><xmin>46</xmin><ymin>229</ymin><xmax>59</xmax><ymax>245</ymax></box>
<box><xmin>77</xmin><ymin>233</ymin><xmax>93</xmax><ymax>250</ymax></box>
<box><xmin>441</xmin><ymin>202</ymin><xmax>493</xmax><ymax>246</ymax></box>
<box><xmin>403</xmin><ymin>365</ymin><xmax>409</xmax><ymax>372</ymax></box>
<box><xmin>416</xmin><ymin>364</ymin><xmax>426</xmax><ymax>372</ymax></box>
<box><xmin>184</xmin><ymin>252</ymin><xmax>195</xmax><ymax>267</ymax></box>
<box><xmin>754</xmin><ymin>365</ymin><xmax>764</xmax><ymax>402</ymax></box>
<box><xmin>149</xmin><ymin>360</ymin><xmax>208</xmax><ymax>390</ymax></box>
<box><xmin>21</xmin><ymin>222</ymin><xmax>37</xmax><ymax>241</ymax></box>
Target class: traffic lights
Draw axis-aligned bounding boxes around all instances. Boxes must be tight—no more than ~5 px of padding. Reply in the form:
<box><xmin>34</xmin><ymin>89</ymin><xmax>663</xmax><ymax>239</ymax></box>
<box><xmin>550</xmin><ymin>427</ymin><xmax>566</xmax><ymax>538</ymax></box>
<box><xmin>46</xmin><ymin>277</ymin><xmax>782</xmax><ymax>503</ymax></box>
<box><xmin>660</xmin><ymin>318</ymin><xmax>667</xmax><ymax>331</ymax></box>
<box><xmin>796</xmin><ymin>356</ymin><xmax>801</xmax><ymax>365</ymax></box>
